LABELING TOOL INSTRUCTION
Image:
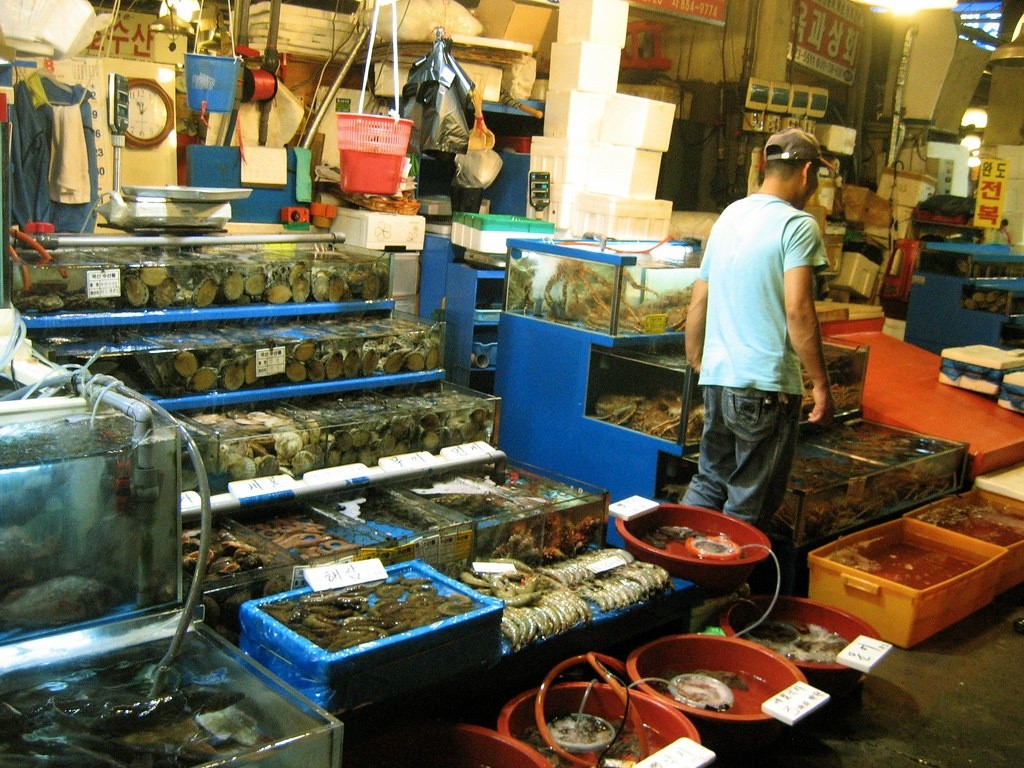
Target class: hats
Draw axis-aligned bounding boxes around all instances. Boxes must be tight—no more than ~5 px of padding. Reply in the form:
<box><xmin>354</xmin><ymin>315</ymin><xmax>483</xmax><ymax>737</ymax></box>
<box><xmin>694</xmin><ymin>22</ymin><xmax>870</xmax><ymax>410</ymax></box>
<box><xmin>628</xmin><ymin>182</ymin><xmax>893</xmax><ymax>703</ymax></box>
<box><xmin>764</xmin><ymin>127</ymin><xmax>835</xmax><ymax>171</ymax></box>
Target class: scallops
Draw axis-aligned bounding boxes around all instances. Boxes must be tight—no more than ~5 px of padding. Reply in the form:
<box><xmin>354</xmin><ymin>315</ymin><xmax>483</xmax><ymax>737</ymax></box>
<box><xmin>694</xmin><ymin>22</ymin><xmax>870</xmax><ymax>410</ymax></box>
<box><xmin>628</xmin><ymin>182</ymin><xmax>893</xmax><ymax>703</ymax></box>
<box><xmin>187</xmin><ymin>406</ymin><xmax>326</xmax><ymax>479</ymax></box>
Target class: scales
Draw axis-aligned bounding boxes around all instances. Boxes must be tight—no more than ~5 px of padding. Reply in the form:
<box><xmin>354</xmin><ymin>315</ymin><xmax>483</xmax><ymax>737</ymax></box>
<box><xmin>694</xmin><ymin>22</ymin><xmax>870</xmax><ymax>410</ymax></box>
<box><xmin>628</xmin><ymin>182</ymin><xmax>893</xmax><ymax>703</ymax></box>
<box><xmin>94</xmin><ymin>72</ymin><xmax>232</xmax><ymax>230</ymax></box>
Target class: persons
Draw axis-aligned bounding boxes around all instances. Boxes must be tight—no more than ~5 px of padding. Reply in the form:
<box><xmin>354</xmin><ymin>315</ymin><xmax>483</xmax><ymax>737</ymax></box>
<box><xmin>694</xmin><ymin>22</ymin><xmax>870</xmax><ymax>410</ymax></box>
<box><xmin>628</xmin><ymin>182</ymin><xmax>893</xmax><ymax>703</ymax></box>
<box><xmin>993</xmin><ymin>219</ymin><xmax>1011</xmax><ymax>244</ymax></box>
<box><xmin>685</xmin><ymin>128</ymin><xmax>835</xmax><ymax>577</ymax></box>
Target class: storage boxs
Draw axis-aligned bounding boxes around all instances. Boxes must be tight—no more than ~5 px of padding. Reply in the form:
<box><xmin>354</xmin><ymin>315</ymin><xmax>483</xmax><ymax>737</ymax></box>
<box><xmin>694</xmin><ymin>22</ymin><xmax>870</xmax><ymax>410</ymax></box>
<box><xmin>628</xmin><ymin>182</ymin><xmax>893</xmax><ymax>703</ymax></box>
<box><xmin>810</xmin><ymin>124</ymin><xmax>936</xmax><ymax>303</ymax></box>
<box><xmin>473</xmin><ymin>1</ymin><xmax>560</xmax><ymax>68</ymax></box>
<box><xmin>327</xmin><ymin>207</ymin><xmax>427</xmax><ymax>250</ymax></box>
<box><xmin>803</xmin><ymin>459</ymin><xmax>1024</xmax><ymax>650</ymax></box>
<box><xmin>229</xmin><ymin>558</ymin><xmax>506</xmax><ymax>716</ymax></box>
<box><xmin>566</xmin><ymin>82</ymin><xmax>695</xmax><ymax>245</ymax></box>
<box><xmin>448</xmin><ymin>211</ymin><xmax>557</xmax><ymax>253</ymax></box>
<box><xmin>373</xmin><ymin>62</ymin><xmax>503</xmax><ymax>101</ymax></box>
<box><xmin>938</xmin><ymin>342</ymin><xmax>1024</xmax><ymax>414</ymax></box>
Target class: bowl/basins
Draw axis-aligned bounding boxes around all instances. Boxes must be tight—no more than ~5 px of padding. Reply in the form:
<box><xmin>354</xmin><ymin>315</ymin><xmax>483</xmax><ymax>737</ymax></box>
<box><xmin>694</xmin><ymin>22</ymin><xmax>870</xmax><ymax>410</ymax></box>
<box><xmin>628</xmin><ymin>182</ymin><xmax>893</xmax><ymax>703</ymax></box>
<box><xmin>342</xmin><ymin>721</ymin><xmax>552</xmax><ymax>768</ymax></box>
<box><xmin>719</xmin><ymin>594</ymin><xmax>882</xmax><ymax>694</ymax></box>
<box><xmin>614</xmin><ymin>502</ymin><xmax>772</xmax><ymax>598</ymax></box>
<box><xmin>625</xmin><ymin>633</ymin><xmax>810</xmax><ymax>751</ymax></box>
<box><xmin>496</xmin><ymin>681</ymin><xmax>702</xmax><ymax>768</ymax></box>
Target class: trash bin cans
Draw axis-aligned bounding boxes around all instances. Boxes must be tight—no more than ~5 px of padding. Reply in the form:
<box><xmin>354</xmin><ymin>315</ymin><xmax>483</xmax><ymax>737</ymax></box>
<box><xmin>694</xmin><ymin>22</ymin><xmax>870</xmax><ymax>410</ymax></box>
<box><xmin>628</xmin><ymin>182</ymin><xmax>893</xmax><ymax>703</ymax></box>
<box><xmin>184</xmin><ymin>52</ymin><xmax>242</xmax><ymax>113</ymax></box>
<box><xmin>335</xmin><ymin>112</ymin><xmax>414</xmax><ymax>195</ymax></box>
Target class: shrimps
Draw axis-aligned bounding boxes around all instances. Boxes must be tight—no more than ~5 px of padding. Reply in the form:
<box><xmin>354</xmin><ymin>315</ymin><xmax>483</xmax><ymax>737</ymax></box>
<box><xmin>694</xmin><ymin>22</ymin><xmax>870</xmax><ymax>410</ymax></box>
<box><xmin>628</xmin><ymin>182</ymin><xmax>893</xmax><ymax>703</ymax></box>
<box><xmin>263</xmin><ymin>576</ymin><xmax>472</xmax><ymax>650</ymax></box>
<box><xmin>460</xmin><ymin>547</ymin><xmax>674</xmax><ymax>645</ymax></box>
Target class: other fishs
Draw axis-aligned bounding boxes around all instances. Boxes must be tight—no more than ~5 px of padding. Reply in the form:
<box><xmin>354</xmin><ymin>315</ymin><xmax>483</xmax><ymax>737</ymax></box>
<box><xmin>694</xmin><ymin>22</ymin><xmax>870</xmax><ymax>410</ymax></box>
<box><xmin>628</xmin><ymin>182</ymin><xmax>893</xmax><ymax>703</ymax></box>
<box><xmin>0</xmin><ymin>661</ymin><xmax>244</xmax><ymax>768</ymax></box>
<box><xmin>0</xmin><ymin>470</ymin><xmax>135</xmax><ymax>630</ymax></box>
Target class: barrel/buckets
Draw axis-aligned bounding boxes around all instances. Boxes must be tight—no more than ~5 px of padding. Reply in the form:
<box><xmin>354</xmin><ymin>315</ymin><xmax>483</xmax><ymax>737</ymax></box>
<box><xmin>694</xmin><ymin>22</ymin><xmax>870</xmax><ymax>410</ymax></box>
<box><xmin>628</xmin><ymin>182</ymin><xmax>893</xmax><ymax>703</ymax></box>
<box><xmin>334</xmin><ymin>111</ymin><xmax>414</xmax><ymax>196</ymax></box>
<box><xmin>183</xmin><ymin>52</ymin><xmax>242</xmax><ymax>112</ymax></box>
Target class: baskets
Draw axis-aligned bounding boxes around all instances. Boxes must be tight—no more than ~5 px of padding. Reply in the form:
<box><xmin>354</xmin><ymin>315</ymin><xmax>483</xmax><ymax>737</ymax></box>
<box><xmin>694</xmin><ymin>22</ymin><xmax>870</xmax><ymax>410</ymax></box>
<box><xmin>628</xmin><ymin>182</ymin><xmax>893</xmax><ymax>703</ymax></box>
<box><xmin>336</xmin><ymin>113</ymin><xmax>415</xmax><ymax>156</ymax></box>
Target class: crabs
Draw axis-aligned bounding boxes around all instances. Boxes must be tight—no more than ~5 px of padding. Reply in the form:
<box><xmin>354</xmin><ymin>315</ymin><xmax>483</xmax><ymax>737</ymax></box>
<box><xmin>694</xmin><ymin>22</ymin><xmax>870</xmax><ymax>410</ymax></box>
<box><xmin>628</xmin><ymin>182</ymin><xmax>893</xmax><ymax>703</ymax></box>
<box><xmin>774</xmin><ymin>422</ymin><xmax>956</xmax><ymax>524</ymax></box>
<box><xmin>597</xmin><ymin>351</ymin><xmax>859</xmax><ymax>443</ymax></box>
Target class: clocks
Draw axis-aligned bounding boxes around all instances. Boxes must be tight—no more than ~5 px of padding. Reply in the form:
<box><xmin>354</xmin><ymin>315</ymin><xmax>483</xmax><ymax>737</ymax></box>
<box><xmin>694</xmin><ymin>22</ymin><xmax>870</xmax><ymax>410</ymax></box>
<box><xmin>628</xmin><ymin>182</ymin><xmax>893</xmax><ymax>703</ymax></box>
<box><xmin>120</xmin><ymin>78</ymin><xmax>175</xmax><ymax>148</ymax></box>
